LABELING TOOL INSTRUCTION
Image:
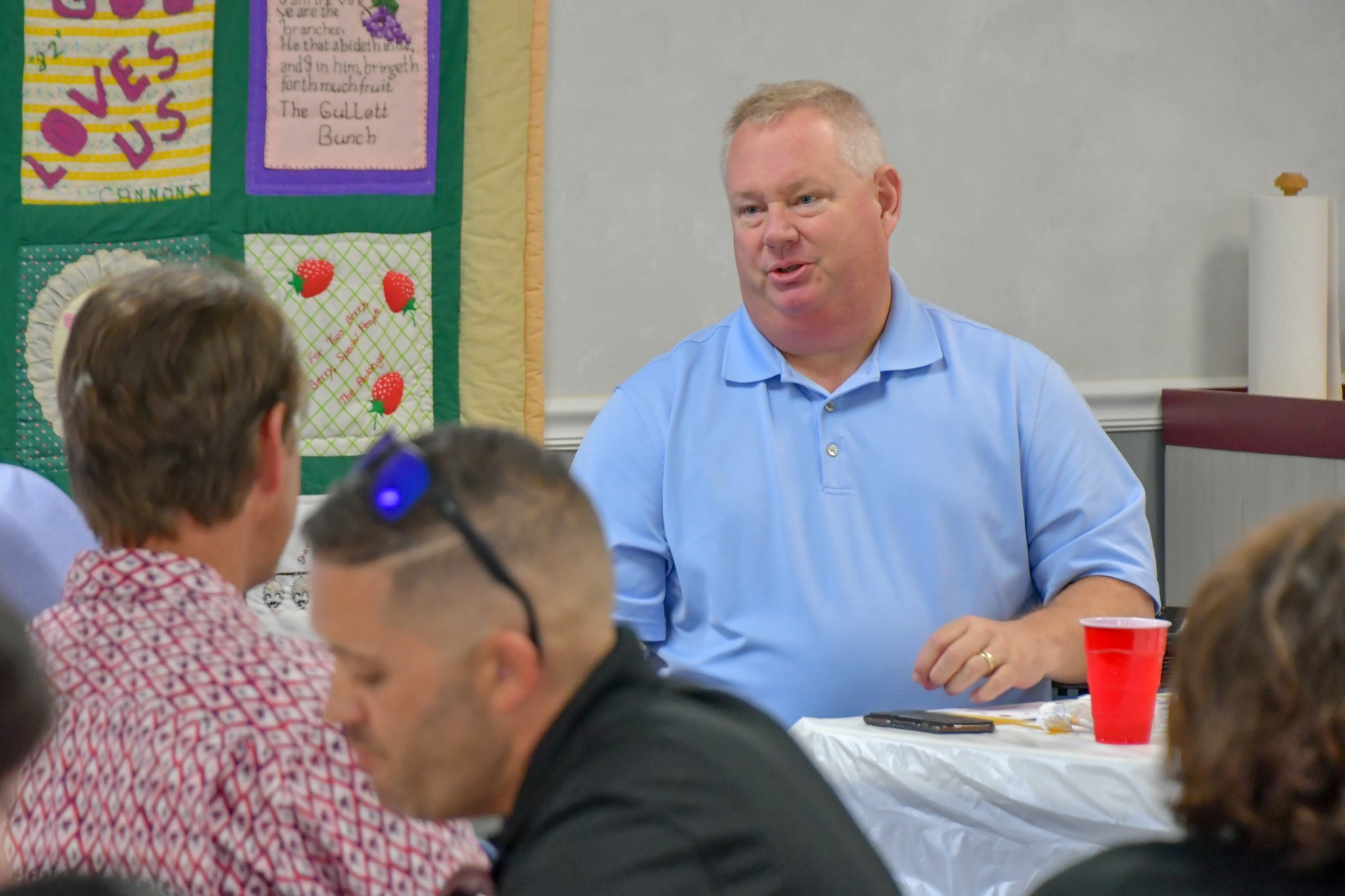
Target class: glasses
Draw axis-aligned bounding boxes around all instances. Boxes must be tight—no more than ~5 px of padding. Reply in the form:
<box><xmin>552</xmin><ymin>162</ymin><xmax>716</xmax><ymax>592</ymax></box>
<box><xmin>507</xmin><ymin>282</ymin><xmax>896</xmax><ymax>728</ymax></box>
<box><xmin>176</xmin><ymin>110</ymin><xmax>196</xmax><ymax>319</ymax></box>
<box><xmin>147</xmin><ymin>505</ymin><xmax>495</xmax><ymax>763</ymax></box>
<box><xmin>357</xmin><ymin>429</ymin><xmax>542</xmax><ymax>664</ymax></box>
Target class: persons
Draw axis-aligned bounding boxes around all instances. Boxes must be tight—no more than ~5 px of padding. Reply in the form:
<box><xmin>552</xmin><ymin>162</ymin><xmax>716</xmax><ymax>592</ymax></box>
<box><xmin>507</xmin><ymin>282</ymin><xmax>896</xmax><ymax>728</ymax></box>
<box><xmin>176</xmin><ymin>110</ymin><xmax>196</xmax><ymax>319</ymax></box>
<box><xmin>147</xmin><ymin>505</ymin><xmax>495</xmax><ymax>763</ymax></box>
<box><xmin>299</xmin><ymin>426</ymin><xmax>905</xmax><ymax>895</ymax></box>
<box><xmin>4</xmin><ymin>869</ymin><xmax>169</xmax><ymax>895</ymax></box>
<box><xmin>1029</xmin><ymin>494</ymin><xmax>1344</xmax><ymax>896</ymax></box>
<box><xmin>565</xmin><ymin>79</ymin><xmax>1164</xmax><ymax>729</ymax></box>
<box><xmin>4</xmin><ymin>256</ymin><xmax>495</xmax><ymax>895</ymax></box>
<box><xmin>0</xmin><ymin>587</ymin><xmax>52</xmax><ymax>896</ymax></box>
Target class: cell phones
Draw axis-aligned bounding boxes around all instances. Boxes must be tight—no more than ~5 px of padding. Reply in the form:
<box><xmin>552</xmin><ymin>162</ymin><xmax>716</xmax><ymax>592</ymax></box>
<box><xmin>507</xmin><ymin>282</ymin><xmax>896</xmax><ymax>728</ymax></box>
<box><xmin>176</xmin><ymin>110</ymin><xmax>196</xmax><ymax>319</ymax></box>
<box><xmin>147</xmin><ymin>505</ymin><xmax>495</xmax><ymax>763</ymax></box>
<box><xmin>864</xmin><ymin>710</ymin><xmax>992</xmax><ymax>732</ymax></box>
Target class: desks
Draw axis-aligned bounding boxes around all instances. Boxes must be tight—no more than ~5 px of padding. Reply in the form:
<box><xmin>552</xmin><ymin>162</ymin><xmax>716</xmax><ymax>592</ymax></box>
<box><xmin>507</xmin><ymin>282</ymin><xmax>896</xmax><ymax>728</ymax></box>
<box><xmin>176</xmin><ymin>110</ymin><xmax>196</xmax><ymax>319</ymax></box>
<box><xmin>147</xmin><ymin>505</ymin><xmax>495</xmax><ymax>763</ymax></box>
<box><xmin>792</xmin><ymin>689</ymin><xmax>1191</xmax><ymax>895</ymax></box>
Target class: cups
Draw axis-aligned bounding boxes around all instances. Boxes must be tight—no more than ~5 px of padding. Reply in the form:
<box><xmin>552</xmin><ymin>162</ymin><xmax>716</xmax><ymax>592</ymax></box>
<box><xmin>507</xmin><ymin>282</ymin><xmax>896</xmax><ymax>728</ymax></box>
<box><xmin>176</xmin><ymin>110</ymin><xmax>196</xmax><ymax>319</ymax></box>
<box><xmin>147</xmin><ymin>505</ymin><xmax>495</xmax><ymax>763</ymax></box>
<box><xmin>1080</xmin><ymin>617</ymin><xmax>1171</xmax><ymax>747</ymax></box>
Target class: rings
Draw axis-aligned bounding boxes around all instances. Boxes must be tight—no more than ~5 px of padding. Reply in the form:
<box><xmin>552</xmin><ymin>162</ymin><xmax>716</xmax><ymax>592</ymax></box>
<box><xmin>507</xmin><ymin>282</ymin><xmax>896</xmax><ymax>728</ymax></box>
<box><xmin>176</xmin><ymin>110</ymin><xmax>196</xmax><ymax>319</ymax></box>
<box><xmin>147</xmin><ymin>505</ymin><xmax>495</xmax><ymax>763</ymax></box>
<box><xmin>979</xmin><ymin>651</ymin><xmax>996</xmax><ymax>675</ymax></box>
